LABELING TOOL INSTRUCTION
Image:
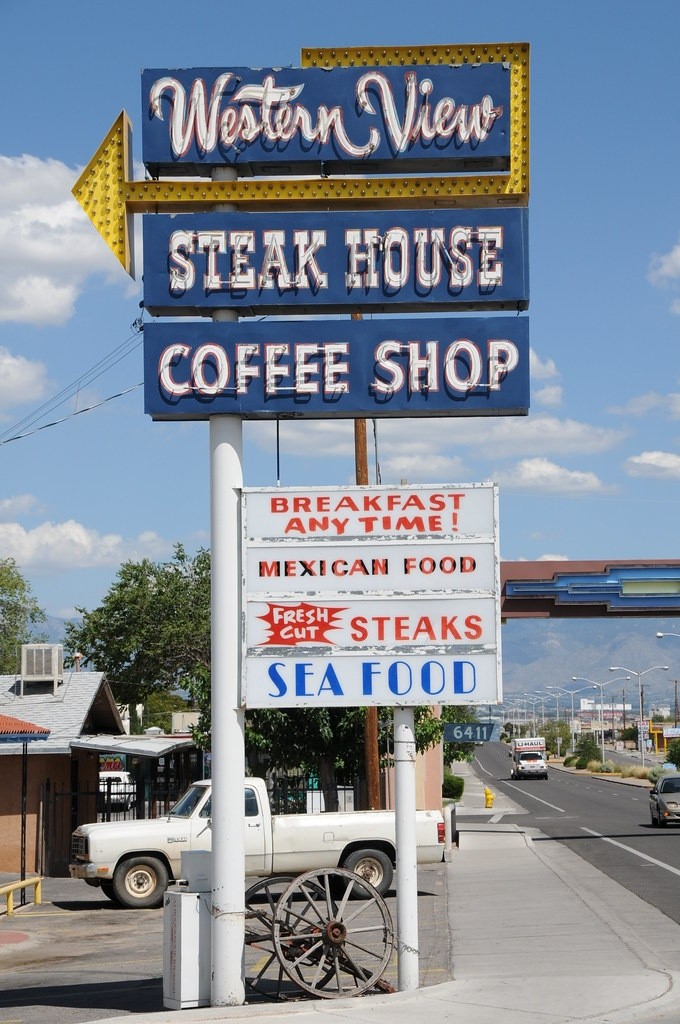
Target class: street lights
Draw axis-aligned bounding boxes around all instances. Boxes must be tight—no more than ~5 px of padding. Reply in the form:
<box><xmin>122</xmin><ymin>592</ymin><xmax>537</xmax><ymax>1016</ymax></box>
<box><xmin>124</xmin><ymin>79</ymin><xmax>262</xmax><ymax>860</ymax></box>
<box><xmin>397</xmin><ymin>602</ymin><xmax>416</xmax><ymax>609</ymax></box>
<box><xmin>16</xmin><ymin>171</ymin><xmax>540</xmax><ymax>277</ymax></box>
<box><xmin>545</xmin><ymin>685</ymin><xmax>597</xmax><ymax>758</ymax></box>
<box><xmin>499</xmin><ymin>690</ymin><xmax>573</xmax><ymax>759</ymax></box>
<box><xmin>572</xmin><ymin>676</ymin><xmax>631</xmax><ymax>766</ymax></box>
<box><xmin>608</xmin><ymin>666</ymin><xmax>670</xmax><ymax>767</ymax></box>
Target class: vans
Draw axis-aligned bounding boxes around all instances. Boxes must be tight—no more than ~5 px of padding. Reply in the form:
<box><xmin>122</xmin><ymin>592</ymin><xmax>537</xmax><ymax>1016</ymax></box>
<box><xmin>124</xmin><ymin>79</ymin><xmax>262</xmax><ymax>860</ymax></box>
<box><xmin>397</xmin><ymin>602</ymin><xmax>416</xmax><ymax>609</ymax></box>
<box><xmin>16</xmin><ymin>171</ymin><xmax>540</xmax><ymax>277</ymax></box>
<box><xmin>509</xmin><ymin>735</ymin><xmax>550</xmax><ymax>781</ymax></box>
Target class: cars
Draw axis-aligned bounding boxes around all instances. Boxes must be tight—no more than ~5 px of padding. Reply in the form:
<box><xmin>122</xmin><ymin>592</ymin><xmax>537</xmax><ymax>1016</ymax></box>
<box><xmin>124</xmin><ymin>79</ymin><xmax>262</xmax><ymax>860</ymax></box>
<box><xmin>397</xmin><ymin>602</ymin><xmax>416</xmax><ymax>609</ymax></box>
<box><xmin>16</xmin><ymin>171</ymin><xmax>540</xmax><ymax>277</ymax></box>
<box><xmin>98</xmin><ymin>771</ymin><xmax>137</xmax><ymax>811</ymax></box>
<box><xmin>649</xmin><ymin>774</ymin><xmax>680</xmax><ymax>827</ymax></box>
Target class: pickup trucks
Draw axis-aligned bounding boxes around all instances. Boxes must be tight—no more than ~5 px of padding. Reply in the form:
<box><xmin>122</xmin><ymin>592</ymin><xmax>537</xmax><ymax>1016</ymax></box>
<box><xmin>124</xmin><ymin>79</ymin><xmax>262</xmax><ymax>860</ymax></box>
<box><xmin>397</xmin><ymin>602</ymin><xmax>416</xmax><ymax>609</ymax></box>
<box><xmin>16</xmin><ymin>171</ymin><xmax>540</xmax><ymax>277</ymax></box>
<box><xmin>65</xmin><ymin>774</ymin><xmax>446</xmax><ymax>913</ymax></box>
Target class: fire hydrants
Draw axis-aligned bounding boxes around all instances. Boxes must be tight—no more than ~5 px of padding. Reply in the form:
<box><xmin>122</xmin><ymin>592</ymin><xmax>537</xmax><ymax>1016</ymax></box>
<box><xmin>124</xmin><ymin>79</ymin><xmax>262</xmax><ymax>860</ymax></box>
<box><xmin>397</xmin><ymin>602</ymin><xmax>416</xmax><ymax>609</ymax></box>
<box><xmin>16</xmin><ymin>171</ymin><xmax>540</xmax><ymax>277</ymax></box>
<box><xmin>485</xmin><ymin>789</ymin><xmax>496</xmax><ymax>808</ymax></box>
